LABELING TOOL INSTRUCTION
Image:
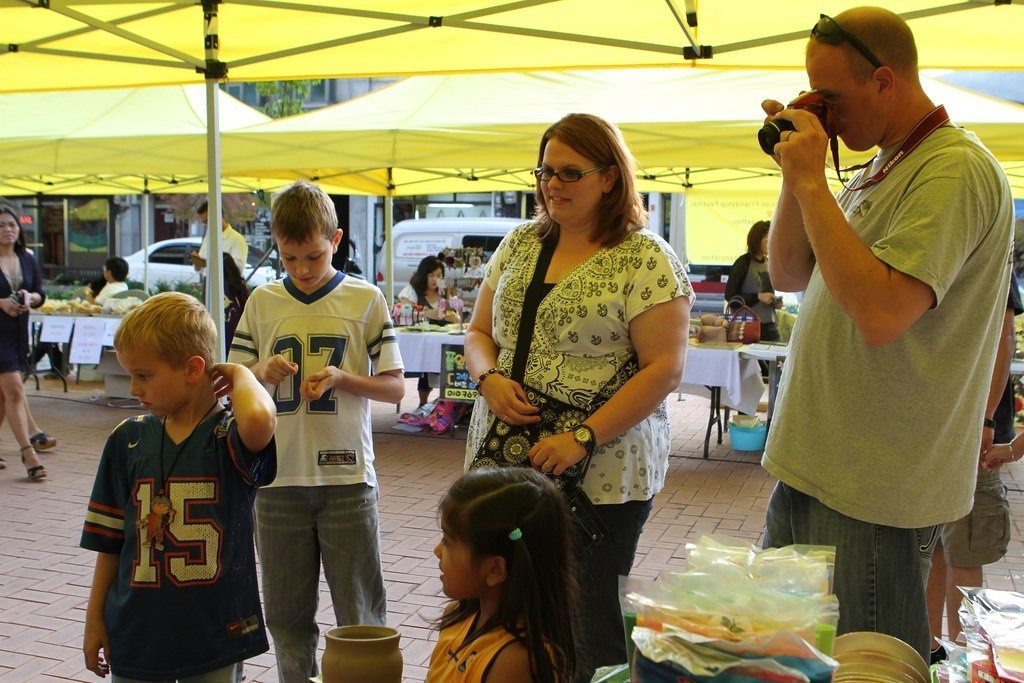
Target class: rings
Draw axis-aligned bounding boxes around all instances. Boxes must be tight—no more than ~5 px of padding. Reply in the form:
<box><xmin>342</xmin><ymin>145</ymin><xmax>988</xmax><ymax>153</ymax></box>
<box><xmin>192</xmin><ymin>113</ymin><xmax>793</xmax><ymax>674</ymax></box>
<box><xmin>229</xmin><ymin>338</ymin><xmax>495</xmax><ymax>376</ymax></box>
<box><xmin>786</xmin><ymin>131</ymin><xmax>792</xmax><ymax>141</ymax></box>
<box><xmin>546</xmin><ymin>460</ymin><xmax>554</xmax><ymax>467</ymax></box>
<box><xmin>983</xmin><ymin>450</ymin><xmax>987</xmax><ymax>454</ymax></box>
<box><xmin>503</xmin><ymin>416</ymin><xmax>507</xmax><ymax>421</ymax></box>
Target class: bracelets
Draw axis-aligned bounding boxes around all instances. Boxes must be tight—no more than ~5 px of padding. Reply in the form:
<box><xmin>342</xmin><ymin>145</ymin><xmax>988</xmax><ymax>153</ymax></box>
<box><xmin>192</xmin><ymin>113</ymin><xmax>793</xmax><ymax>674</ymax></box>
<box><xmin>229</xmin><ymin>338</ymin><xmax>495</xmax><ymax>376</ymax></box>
<box><xmin>85</xmin><ymin>291</ymin><xmax>90</xmax><ymax>295</ymax></box>
<box><xmin>1009</xmin><ymin>443</ymin><xmax>1014</xmax><ymax>459</ymax></box>
<box><xmin>476</xmin><ymin>368</ymin><xmax>504</xmax><ymax>396</ymax></box>
<box><xmin>984</xmin><ymin>418</ymin><xmax>996</xmax><ymax>429</ymax></box>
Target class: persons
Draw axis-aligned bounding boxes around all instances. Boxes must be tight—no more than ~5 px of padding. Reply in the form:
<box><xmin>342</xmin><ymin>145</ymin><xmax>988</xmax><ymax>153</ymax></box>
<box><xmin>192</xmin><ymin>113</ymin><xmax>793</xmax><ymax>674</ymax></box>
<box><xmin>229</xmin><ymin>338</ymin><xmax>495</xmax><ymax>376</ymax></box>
<box><xmin>761</xmin><ymin>6</ymin><xmax>1015</xmax><ymax>668</ymax></box>
<box><xmin>227</xmin><ymin>183</ymin><xmax>406</xmax><ymax>683</ymax></box>
<box><xmin>984</xmin><ymin>432</ymin><xmax>1024</xmax><ymax>472</ymax></box>
<box><xmin>79</xmin><ymin>291</ymin><xmax>278</xmax><ymax>683</ymax></box>
<box><xmin>926</xmin><ymin>274</ymin><xmax>1015</xmax><ymax>666</ymax></box>
<box><xmin>724</xmin><ymin>220</ymin><xmax>782</xmax><ymax>383</ymax></box>
<box><xmin>192</xmin><ymin>202</ymin><xmax>248</xmax><ymax>304</ymax></box>
<box><xmin>30</xmin><ymin>257</ymin><xmax>129</xmax><ymax>380</ymax></box>
<box><xmin>397</xmin><ymin>256</ymin><xmax>460</xmax><ymax>408</ymax></box>
<box><xmin>463</xmin><ymin>113</ymin><xmax>696</xmax><ymax>683</ymax></box>
<box><xmin>422</xmin><ymin>466</ymin><xmax>578</xmax><ymax>683</ymax></box>
<box><xmin>0</xmin><ymin>205</ymin><xmax>57</xmax><ymax>479</ymax></box>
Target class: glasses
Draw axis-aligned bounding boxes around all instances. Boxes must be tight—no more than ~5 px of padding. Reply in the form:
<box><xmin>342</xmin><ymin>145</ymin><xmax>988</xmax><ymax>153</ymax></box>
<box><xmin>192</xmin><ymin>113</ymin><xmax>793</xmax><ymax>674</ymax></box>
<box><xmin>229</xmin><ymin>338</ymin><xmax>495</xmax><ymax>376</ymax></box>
<box><xmin>534</xmin><ymin>161</ymin><xmax>617</xmax><ymax>182</ymax></box>
<box><xmin>810</xmin><ymin>14</ymin><xmax>883</xmax><ymax>68</ymax></box>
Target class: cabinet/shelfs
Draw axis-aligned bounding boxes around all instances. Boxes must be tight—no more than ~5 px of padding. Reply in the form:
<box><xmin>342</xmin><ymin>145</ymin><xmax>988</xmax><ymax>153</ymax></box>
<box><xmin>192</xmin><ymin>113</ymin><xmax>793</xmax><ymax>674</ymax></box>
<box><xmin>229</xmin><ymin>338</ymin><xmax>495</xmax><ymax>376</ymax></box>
<box><xmin>443</xmin><ymin>276</ymin><xmax>485</xmax><ymax>328</ymax></box>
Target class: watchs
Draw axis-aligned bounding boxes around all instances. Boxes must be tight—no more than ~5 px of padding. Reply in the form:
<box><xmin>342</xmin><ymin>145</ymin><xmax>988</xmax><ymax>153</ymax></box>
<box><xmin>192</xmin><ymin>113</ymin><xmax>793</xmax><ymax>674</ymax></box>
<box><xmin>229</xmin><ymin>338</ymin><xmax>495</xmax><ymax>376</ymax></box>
<box><xmin>574</xmin><ymin>428</ymin><xmax>593</xmax><ymax>451</ymax></box>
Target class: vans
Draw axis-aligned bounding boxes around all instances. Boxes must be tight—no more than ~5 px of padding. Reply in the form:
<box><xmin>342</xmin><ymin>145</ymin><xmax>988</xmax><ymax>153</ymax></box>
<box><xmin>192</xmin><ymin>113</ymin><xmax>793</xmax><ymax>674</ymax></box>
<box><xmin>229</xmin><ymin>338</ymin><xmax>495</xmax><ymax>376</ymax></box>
<box><xmin>375</xmin><ymin>215</ymin><xmax>541</xmax><ymax>299</ymax></box>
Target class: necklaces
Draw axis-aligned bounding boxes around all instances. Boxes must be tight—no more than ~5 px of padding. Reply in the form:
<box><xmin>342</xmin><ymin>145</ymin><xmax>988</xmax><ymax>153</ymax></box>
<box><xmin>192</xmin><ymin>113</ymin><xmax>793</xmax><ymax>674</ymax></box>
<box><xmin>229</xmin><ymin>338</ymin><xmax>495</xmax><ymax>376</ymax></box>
<box><xmin>136</xmin><ymin>399</ymin><xmax>218</xmax><ymax>551</ymax></box>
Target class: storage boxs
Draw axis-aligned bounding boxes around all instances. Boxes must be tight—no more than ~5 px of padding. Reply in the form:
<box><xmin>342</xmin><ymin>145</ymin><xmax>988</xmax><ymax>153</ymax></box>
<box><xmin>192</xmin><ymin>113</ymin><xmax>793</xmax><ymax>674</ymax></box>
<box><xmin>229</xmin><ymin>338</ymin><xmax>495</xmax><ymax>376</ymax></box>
<box><xmin>96</xmin><ymin>347</ymin><xmax>139</xmax><ymax>401</ymax></box>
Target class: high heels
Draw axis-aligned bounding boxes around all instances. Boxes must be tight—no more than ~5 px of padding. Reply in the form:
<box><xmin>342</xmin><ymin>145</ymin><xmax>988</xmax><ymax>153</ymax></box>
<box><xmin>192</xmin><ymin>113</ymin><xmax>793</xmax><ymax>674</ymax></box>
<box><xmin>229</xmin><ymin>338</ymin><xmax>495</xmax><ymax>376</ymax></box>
<box><xmin>20</xmin><ymin>445</ymin><xmax>46</xmax><ymax>480</ymax></box>
<box><xmin>0</xmin><ymin>458</ymin><xmax>7</xmax><ymax>469</ymax></box>
<box><xmin>30</xmin><ymin>432</ymin><xmax>56</xmax><ymax>451</ymax></box>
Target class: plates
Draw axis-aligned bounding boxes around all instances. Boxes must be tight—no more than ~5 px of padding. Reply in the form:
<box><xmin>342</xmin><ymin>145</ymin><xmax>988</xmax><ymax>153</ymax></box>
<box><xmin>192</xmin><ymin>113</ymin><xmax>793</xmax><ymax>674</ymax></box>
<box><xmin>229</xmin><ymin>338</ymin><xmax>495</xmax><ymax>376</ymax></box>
<box><xmin>832</xmin><ymin>632</ymin><xmax>931</xmax><ymax>683</ymax></box>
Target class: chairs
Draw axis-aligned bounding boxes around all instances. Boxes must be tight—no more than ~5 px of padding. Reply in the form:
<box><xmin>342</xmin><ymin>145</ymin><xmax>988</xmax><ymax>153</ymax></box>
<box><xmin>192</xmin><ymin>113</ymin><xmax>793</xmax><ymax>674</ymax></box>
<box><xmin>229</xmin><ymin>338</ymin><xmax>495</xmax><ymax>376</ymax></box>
<box><xmin>114</xmin><ymin>289</ymin><xmax>149</xmax><ymax>304</ymax></box>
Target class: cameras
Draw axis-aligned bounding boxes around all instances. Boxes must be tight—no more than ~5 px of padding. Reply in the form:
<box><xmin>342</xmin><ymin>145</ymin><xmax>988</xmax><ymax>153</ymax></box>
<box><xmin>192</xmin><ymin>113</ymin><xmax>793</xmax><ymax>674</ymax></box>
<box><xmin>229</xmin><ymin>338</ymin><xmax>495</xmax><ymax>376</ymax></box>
<box><xmin>757</xmin><ymin>88</ymin><xmax>841</xmax><ymax>156</ymax></box>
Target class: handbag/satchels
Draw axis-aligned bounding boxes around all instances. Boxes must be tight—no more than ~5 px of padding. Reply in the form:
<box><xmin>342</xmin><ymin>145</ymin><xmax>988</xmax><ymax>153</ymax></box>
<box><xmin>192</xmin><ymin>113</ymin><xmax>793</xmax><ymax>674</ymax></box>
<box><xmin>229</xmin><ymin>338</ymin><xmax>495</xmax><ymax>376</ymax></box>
<box><xmin>716</xmin><ymin>295</ymin><xmax>761</xmax><ymax>344</ymax></box>
<box><xmin>468</xmin><ymin>383</ymin><xmax>592</xmax><ymax>506</ymax></box>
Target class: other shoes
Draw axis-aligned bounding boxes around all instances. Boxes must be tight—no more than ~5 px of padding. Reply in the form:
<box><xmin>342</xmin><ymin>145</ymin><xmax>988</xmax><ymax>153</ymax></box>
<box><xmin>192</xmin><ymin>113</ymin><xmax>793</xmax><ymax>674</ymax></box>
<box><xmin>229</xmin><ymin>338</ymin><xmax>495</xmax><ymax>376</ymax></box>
<box><xmin>43</xmin><ymin>370</ymin><xmax>66</xmax><ymax>380</ymax></box>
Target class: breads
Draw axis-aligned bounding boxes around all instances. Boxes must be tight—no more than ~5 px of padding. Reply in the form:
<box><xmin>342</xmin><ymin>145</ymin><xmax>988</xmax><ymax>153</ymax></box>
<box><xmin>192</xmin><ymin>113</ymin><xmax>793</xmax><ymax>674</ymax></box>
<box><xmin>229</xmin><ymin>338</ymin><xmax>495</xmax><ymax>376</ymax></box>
<box><xmin>40</xmin><ymin>300</ymin><xmax>104</xmax><ymax>314</ymax></box>
<box><xmin>700</xmin><ymin>315</ymin><xmax>728</xmax><ymax>327</ymax></box>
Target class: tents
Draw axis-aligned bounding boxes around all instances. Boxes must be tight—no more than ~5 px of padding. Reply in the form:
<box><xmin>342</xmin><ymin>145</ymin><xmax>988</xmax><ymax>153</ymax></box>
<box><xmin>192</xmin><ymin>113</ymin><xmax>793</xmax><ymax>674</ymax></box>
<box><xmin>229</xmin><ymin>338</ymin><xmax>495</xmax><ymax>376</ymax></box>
<box><xmin>0</xmin><ymin>68</ymin><xmax>1024</xmax><ymax>399</ymax></box>
<box><xmin>0</xmin><ymin>0</ymin><xmax>1024</xmax><ymax>364</ymax></box>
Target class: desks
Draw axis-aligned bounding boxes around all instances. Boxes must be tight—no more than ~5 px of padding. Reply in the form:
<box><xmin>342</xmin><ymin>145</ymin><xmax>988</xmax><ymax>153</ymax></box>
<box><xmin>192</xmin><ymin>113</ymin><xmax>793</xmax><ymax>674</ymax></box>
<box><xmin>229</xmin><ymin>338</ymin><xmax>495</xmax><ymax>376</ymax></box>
<box><xmin>383</xmin><ymin>322</ymin><xmax>739</xmax><ymax>459</ymax></box>
<box><xmin>12</xmin><ymin>307</ymin><xmax>146</xmax><ymax>392</ymax></box>
<box><xmin>740</xmin><ymin>340</ymin><xmax>793</xmax><ymax>451</ymax></box>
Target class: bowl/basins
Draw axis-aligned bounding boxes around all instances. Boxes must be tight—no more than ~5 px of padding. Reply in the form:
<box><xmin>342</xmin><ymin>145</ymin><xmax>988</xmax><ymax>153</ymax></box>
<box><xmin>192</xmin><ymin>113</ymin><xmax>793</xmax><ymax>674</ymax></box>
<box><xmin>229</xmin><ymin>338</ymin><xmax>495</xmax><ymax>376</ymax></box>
<box><xmin>727</xmin><ymin>424</ymin><xmax>766</xmax><ymax>451</ymax></box>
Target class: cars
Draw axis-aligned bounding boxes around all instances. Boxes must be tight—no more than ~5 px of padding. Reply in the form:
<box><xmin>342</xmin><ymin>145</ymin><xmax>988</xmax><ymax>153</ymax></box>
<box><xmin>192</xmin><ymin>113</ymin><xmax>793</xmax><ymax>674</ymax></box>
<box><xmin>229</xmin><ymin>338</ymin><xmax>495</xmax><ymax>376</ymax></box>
<box><xmin>121</xmin><ymin>236</ymin><xmax>285</xmax><ymax>294</ymax></box>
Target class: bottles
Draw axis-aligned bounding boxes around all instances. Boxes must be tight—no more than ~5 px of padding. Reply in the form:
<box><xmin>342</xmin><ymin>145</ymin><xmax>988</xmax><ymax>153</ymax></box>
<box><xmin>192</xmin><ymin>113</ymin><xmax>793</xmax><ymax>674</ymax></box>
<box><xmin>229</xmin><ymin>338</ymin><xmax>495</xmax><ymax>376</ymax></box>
<box><xmin>322</xmin><ymin>626</ymin><xmax>403</xmax><ymax>683</ymax></box>
